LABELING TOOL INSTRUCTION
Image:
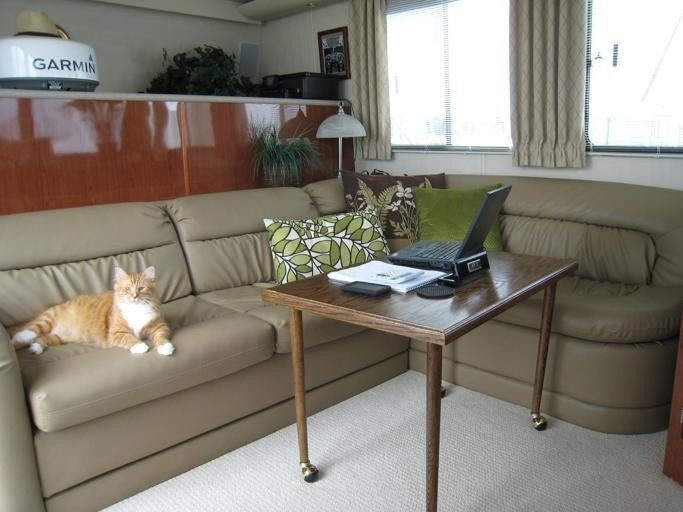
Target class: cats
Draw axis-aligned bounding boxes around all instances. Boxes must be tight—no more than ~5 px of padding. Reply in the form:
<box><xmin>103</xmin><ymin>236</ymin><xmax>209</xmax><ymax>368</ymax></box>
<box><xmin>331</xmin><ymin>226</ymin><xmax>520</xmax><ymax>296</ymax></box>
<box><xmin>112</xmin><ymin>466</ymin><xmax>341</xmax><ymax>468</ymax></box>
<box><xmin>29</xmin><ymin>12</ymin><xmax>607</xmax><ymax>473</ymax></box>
<box><xmin>5</xmin><ymin>266</ymin><xmax>175</xmax><ymax>355</ymax></box>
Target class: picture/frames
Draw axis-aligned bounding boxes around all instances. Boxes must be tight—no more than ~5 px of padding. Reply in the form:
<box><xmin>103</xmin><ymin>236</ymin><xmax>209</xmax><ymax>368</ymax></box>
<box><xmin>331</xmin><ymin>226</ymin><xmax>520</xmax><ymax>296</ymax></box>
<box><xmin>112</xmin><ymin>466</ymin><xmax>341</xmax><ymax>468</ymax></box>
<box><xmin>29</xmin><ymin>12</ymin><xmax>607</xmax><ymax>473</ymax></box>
<box><xmin>316</xmin><ymin>25</ymin><xmax>353</xmax><ymax>81</ymax></box>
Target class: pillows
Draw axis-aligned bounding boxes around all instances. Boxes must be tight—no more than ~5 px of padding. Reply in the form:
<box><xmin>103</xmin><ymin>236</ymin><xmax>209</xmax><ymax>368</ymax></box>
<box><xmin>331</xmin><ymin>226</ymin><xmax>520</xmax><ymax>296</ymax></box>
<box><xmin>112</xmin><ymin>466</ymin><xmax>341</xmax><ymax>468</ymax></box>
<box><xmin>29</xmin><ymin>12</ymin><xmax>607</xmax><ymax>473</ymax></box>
<box><xmin>407</xmin><ymin>183</ymin><xmax>505</xmax><ymax>250</ymax></box>
<box><xmin>262</xmin><ymin>210</ymin><xmax>392</xmax><ymax>287</ymax></box>
<box><xmin>337</xmin><ymin>171</ymin><xmax>445</xmax><ymax>238</ymax></box>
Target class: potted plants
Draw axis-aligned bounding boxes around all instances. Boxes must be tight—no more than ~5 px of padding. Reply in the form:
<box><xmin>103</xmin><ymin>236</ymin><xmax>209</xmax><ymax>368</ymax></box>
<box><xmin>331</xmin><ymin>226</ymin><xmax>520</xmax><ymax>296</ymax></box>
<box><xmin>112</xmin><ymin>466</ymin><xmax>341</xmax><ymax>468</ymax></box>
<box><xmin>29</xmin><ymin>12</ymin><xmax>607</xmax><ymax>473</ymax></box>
<box><xmin>245</xmin><ymin>117</ymin><xmax>314</xmax><ymax>194</ymax></box>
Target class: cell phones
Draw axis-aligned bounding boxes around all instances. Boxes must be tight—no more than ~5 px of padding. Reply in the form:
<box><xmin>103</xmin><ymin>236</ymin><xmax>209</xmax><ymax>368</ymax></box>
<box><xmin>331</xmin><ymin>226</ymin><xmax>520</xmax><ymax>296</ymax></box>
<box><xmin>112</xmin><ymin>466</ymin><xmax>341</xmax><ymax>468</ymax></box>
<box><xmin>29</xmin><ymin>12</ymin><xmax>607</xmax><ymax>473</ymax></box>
<box><xmin>344</xmin><ymin>281</ymin><xmax>390</xmax><ymax>297</ymax></box>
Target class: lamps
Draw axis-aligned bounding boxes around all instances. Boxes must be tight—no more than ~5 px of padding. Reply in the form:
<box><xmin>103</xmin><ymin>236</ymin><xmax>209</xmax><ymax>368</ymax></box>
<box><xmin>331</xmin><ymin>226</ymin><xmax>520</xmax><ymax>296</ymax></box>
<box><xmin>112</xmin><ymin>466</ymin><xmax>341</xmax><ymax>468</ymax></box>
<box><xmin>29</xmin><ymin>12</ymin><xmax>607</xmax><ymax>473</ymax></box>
<box><xmin>316</xmin><ymin>101</ymin><xmax>368</xmax><ymax>178</ymax></box>
<box><xmin>274</xmin><ymin>106</ymin><xmax>322</xmax><ymax>189</ymax></box>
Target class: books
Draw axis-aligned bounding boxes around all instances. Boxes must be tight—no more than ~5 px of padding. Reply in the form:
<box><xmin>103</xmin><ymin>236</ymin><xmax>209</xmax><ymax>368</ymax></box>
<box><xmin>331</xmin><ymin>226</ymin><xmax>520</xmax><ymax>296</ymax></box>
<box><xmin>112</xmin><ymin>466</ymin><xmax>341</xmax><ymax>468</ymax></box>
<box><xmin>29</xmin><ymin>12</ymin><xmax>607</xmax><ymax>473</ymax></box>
<box><xmin>326</xmin><ymin>257</ymin><xmax>451</xmax><ymax>296</ymax></box>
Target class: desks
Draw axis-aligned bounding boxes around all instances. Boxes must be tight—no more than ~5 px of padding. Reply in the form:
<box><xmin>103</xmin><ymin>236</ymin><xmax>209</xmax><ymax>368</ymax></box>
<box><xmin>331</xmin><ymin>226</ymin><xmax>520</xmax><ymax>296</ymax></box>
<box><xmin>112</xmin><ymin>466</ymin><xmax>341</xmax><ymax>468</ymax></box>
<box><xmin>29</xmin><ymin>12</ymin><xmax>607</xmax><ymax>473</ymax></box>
<box><xmin>260</xmin><ymin>245</ymin><xmax>581</xmax><ymax>512</ymax></box>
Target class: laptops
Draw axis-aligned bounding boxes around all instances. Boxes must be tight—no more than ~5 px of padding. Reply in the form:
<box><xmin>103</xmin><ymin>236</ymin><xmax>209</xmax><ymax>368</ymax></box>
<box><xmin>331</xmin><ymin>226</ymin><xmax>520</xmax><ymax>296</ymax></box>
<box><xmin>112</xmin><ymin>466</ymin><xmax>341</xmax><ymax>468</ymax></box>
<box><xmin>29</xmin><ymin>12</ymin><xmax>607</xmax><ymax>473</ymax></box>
<box><xmin>387</xmin><ymin>185</ymin><xmax>512</xmax><ymax>270</ymax></box>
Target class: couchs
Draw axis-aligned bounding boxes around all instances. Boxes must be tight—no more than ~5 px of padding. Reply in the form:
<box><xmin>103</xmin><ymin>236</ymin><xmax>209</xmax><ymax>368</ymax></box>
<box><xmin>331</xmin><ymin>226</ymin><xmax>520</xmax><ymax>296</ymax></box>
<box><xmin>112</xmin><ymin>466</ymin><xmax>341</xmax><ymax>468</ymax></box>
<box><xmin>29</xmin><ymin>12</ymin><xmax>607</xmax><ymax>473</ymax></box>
<box><xmin>0</xmin><ymin>174</ymin><xmax>683</xmax><ymax>511</ymax></box>
